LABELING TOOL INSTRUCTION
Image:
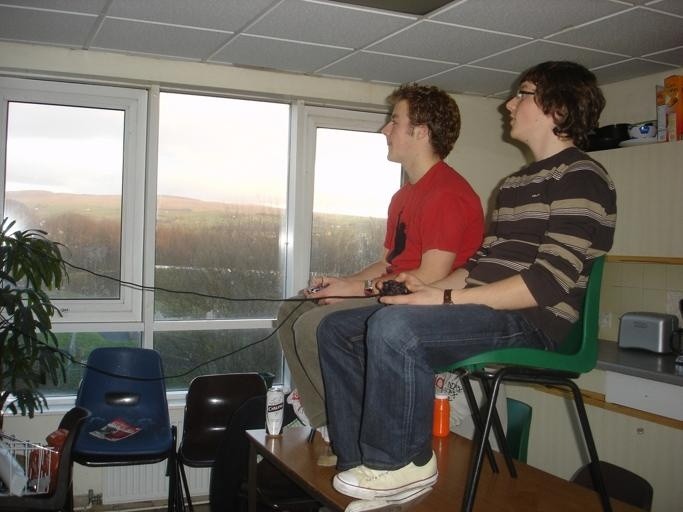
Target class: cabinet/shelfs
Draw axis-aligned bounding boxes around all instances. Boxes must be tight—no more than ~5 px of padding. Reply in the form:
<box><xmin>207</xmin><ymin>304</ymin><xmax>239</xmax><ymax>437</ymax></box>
<box><xmin>503</xmin><ymin>382</ymin><xmax>682</xmax><ymax>512</ymax></box>
<box><xmin>583</xmin><ymin>138</ymin><xmax>682</xmax><ymax>264</ymax></box>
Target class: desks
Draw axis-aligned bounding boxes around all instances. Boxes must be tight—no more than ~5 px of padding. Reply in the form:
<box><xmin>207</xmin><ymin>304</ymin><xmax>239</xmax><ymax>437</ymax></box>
<box><xmin>239</xmin><ymin>423</ymin><xmax>646</xmax><ymax>511</ymax></box>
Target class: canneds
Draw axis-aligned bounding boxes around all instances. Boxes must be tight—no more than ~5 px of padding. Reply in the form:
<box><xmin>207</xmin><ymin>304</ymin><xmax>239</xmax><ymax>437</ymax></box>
<box><xmin>266</xmin><ymin>388</ymin><xmax>285</xmax><ymax>438</ymax></box>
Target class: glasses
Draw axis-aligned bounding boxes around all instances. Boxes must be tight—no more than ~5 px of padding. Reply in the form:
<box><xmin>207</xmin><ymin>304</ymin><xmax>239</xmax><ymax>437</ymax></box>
<box><xmin>515</xmin><ymin>88</ymin><xmax>537</xmax><ymax>97</ymax></box>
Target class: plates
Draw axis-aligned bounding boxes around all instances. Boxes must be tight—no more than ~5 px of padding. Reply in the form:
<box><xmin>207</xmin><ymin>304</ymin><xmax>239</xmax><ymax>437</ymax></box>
<box><xmin>620</xmin><ymin>139</ymin><xmax>656</xmax><ymax>145</ymax></box>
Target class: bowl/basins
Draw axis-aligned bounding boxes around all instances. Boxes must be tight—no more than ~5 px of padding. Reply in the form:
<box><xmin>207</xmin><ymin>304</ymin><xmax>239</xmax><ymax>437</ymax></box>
<box><xmin>629</xmin><ymin>125</ymin><xmax>657</xmax><ymax>139</ymax></box>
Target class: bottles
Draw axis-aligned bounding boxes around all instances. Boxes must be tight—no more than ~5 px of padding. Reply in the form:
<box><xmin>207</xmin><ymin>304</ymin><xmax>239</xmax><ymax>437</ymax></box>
<box><xmin>433</xmin><ymin>437</ymin><xmax>450</xmax><ymax>473</ymax></box>
<box><xmin>433</xmin><ymin>393</ymin><xmax>450</xmax><ymax>437</ymax></box>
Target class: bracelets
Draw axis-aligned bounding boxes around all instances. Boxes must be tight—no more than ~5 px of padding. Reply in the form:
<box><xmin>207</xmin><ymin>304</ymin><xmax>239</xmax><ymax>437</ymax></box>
<box><xmin>442</xmin><ymin>288</ymin><xmax>454</xmax><ymax>305</ymax></box>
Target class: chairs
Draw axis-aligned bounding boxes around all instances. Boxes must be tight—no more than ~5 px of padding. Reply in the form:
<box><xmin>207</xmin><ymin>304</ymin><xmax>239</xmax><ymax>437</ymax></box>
<box><xmin>0</xmin><ymin>405</ymin><xmax>91</xmax><ymax>512</ymax></box>
<box><xmin>169</xmin><ymin>370</ymin><xmax>271</xmax><ymax>512</ymax></box>
<box><xmin>69</xmin><ymin>345</ymin><xmax>181</xmax><ymax>510</ymax></box>
<box><xmin>442</xmin><ymin>246</ymin><xmax>615</xmax><ymax>510</ymax></box>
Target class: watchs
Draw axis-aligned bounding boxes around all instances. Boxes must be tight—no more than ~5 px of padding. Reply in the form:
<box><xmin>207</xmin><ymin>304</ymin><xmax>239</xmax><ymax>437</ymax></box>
<box><xmin>363</xmin><ymin>279</ymin><xmax>375</xmax><ymax>296</ymax></box>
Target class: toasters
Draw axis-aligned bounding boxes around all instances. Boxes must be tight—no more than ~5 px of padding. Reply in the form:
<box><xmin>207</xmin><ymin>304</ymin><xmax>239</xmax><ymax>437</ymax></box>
<box><xmin>615</xmin><ymin>310</ymin><xmax>679</xmax><ymax>356</ymax></box>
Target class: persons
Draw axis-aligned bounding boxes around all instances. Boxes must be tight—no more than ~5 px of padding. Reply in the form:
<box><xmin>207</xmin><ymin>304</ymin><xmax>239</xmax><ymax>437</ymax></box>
<box><xmin>315</xmin><ymin>58</ymin><xmax>616</xmax><ymax>501</ymax></box>
<box><xmin>277</xmin><ymin>80</ymin><xmax>485</xmax><ymax>466</ymax></box>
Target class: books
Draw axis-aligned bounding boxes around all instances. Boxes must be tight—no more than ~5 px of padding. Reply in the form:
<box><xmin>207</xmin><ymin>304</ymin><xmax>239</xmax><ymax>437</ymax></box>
<box><xmin>88</xmin><ymin>419</ymin><xmax>142</xmax><ymax>442</ymax></box>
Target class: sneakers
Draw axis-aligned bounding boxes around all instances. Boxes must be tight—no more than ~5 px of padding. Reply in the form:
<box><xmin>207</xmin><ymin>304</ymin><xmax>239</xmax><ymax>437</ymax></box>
<box><xmin>334</xmin><ymin>447</ymin><xmax>439</xmax><ymax>512</ymax></box>
